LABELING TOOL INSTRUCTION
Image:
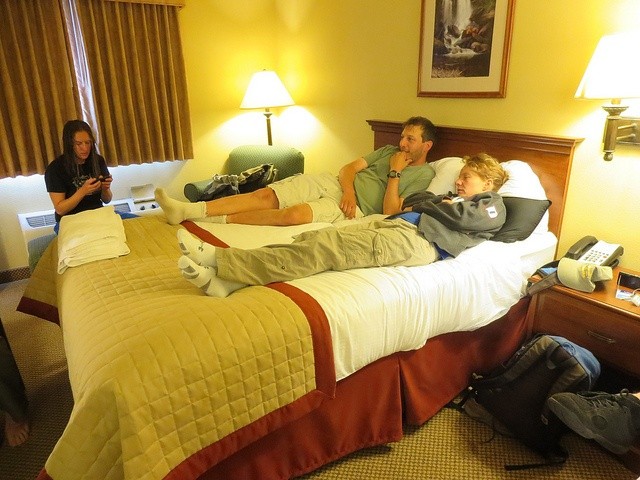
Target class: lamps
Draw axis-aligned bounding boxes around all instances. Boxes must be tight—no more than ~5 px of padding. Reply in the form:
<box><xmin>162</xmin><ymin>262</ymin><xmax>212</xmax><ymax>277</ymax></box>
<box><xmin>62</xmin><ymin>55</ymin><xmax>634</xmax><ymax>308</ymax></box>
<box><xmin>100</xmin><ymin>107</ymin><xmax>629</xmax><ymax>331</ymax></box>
<box><xmin>240</xmin><ymin>68</ymin><xmax>294</xmax><ymax>147</ymax></box>
<box><xmin>577</xmin><ymin>30</ymin><xmax>640</xmax><ymax>159</ymax></box>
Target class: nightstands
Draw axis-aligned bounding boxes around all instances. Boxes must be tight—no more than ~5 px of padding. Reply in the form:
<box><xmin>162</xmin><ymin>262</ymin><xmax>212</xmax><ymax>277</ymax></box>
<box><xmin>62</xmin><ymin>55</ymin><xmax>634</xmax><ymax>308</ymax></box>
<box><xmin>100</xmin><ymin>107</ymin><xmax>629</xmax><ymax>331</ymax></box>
<box><xmin>528</xmin><ymin>259</ymin><xmax>640</xmax><ymax>392</ymax></box>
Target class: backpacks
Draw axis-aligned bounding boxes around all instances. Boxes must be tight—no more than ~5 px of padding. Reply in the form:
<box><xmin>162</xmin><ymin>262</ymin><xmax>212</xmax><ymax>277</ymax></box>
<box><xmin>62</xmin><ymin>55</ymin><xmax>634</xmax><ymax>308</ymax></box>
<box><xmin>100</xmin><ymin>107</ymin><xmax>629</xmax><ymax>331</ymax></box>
<box><xmin>467</xmin><ymin>335</ymin><xmax>601</xmax><ymax>466</ymax></box>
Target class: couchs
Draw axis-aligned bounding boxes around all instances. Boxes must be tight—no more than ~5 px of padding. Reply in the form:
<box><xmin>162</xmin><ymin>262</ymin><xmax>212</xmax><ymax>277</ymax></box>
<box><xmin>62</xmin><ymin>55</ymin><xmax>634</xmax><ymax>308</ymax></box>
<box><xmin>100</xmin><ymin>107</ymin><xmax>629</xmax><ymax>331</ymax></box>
<box><xmin>184</xmin><ymin>146</ymin><xmax>304</xmax><ymax>202</ymax></box>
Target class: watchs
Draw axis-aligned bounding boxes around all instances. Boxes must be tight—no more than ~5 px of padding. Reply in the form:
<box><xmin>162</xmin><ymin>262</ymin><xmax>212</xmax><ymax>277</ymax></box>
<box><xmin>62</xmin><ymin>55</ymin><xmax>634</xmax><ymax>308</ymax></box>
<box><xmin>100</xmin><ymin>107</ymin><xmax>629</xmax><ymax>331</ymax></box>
<box><xmin>386</xmin><ymin>170</ymin><xmax>401</xmax><ymax>178</ymax></box>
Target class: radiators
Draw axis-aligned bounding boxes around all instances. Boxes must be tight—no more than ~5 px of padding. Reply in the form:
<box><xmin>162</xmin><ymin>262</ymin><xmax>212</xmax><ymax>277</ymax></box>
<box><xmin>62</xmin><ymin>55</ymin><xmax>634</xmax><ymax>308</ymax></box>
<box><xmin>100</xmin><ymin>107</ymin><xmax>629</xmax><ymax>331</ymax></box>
<box><xmin>17</xmin><ymin>197</ymin><xmax>165</xmax><ymax>254</ymax></box>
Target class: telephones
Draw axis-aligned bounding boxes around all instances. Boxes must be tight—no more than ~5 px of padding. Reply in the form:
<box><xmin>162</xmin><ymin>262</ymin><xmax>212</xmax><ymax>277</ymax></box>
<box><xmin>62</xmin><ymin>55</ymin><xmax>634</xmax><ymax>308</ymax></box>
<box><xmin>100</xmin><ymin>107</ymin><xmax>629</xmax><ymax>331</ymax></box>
<box><xmin>565</xmin><ymin>235</ymin><xmax>623</xmax><ymax>269</ymax></box>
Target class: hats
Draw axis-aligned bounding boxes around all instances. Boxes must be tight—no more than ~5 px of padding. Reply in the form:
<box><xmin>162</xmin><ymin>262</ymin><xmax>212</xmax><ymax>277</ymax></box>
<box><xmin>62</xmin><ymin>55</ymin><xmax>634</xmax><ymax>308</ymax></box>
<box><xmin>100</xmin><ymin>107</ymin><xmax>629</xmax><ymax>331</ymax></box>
<box><xmin>557</xmin><ymin>258</ymin><xmax>614</xmax><ymax>294</ymax></box>
<box><xmin>526</xmin><ymin>259</ymin><xmax>563</xmax><ymax>296</ymax></box>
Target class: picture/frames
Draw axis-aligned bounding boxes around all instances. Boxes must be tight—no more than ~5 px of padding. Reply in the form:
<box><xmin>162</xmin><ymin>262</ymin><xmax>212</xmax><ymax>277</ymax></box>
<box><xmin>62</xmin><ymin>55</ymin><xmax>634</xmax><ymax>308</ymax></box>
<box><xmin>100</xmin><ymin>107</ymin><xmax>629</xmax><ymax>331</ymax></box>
<box><xmin>418</xmin><ymin>1</ymin><xmax>514</xmax><ymax>100</ymax></box>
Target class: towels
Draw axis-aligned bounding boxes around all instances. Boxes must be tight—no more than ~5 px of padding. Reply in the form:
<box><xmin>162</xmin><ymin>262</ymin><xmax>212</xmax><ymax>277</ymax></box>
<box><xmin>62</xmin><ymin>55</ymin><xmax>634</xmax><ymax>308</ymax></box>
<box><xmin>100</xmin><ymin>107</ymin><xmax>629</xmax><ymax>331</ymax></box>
<box><xmin>54</xmin><ymin>208</ymin><xmax>132</xmax><ymax>276</ymax></box>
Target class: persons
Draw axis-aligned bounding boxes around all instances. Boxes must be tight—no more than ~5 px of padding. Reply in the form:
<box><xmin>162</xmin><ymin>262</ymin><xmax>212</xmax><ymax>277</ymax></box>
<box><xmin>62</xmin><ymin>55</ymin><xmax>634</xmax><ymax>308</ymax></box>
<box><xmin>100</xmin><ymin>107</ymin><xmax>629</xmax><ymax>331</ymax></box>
<box><xmin>43</xmin><ymin>119</ymin><xmax>141</xmax><ymax>237</ymax></box>
<box><xmin>175</xmin><ymin>151</ymin><xmax>509</xmax><ymax>299</ymax></box>
<box><xmin>151</xmin><ymin>115</ymin><xmax>437</xmax><ymax>229</ymax></box>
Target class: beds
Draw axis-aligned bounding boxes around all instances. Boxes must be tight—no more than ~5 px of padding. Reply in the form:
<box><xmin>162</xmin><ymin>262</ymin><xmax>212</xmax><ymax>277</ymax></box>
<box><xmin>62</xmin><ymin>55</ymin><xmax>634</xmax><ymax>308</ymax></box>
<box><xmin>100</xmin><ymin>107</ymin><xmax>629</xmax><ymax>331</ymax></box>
<box><xmin>16</xmin><ymin>117</ymin><xmax>584</xmax><ymax>480</ymax></box>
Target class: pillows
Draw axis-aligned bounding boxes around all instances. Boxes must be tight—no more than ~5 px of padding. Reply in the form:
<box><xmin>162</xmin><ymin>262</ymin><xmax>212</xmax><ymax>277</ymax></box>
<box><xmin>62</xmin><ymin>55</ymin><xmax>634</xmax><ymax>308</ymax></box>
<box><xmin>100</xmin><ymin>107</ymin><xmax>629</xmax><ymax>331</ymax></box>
<box><xmin>425</xmin><ymin>156</ymin><xmax>465</xmax><ymax>196</ymax></box>
<box><xmin>490</xmin><ymin>196</ymin><xmax>552</xmax><ymax>243</ymax></box>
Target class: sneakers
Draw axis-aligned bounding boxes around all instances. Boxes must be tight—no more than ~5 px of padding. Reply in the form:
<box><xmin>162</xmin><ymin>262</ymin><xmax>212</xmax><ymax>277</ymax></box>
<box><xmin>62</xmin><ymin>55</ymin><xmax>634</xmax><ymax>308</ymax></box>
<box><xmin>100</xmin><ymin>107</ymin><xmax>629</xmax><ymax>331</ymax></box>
<box><xmin>541</xmin><ymin>388</ymin><xmax>639</xmax><ymax>460</ymax></box>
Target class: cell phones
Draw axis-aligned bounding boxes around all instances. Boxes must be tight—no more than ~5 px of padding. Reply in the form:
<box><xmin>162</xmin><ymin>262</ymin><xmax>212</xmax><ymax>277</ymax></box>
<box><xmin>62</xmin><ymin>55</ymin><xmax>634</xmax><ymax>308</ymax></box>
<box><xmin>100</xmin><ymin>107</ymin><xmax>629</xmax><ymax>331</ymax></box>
<box><xmin>91</xmin><ymin>176</ymin><xmax>110</xmax><ymax>184</ymax></box>
